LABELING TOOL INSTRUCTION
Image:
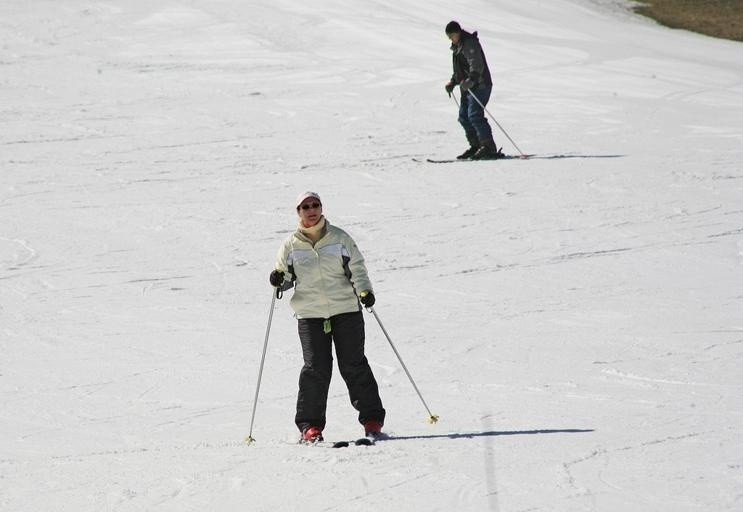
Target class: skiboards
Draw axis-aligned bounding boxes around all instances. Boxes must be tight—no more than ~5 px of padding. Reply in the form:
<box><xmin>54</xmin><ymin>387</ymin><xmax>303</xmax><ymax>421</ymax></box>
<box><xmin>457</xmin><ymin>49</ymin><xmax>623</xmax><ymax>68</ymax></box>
<box><xmin>412</xmin><ymin>155</ymin><xmax>533</xmax><ymax>163</ymax></box>
<box><xmin>280</xmin><ymin>433</ymin><xmax>381</xmax><ymax>448</ymax></box>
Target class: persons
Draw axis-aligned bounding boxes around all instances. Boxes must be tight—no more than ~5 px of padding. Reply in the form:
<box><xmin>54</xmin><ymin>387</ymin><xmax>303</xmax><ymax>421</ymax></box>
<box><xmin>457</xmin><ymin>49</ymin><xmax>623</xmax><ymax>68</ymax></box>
<box><xmin>269</xmin><ymin>192</ymin><xmax>385</xmax><ymax>442</ymax></box>
<box><xmin>445</xmin><ymin>21</ymin><xmax>498</xmax><ymax>160</ymax></box>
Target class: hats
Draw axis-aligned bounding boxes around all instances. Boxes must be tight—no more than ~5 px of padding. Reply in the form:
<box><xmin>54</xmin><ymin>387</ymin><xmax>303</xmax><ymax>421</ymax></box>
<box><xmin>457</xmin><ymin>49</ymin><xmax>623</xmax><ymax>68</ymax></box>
<box><xmin>446</xmin><ymin>21</ymin><xmax>462</xmax><ymax>34</ymax></box>
<box><xmin>296</xmin><ymin>190</ymin><xmax>320</xmax><ymax>209</ymax></box>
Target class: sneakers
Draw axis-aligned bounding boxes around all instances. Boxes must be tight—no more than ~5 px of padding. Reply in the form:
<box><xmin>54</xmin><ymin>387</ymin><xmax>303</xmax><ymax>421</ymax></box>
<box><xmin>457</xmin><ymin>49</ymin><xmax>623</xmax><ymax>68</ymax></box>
<box><xmin>302</xmin><ymin>426</ymin><xmax>322</xmax><ymax>442</ymax></box>
<box><xmin>365</xmin><ymin>419</ymin><xmax>382</xmax><ymax>435</ymax></box>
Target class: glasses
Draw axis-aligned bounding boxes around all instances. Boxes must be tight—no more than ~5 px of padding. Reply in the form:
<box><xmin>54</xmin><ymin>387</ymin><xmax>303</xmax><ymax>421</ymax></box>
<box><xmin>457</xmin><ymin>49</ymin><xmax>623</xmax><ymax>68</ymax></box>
<box><xmin>301</xmin><ymin>202</ymin><xmax>320</xmax><ymax>211</ymax></box>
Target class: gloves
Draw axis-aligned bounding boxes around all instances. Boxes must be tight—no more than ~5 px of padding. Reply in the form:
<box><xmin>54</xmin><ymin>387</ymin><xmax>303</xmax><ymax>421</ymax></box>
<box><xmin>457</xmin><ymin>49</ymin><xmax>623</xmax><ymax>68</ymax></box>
<box><xmin>461</xmin><ymin>79</ymin><xmax>474</xmax><ymax>91</ymax></box>
<box><xmin>270</xmin><ymin>269</ymin><xmax>285</xmax><ymax>288</ymax></box>
<box><xmin>445</xmin><ymin>80</ymin><xmax>455</xmax><ymax>93</ymax></box>
<box><xmin>359</xmin><ymin>292</ymin><xmax>375</xmax><ymax>308</ymax></box>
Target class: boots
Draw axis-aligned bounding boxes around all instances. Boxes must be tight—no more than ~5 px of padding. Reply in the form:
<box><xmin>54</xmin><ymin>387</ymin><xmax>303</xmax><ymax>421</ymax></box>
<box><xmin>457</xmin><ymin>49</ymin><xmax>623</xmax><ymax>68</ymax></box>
<box><xmin>456</xmin><ymin>136</ymin><xmax>497</xmax><ymax>160</ymax></box>
